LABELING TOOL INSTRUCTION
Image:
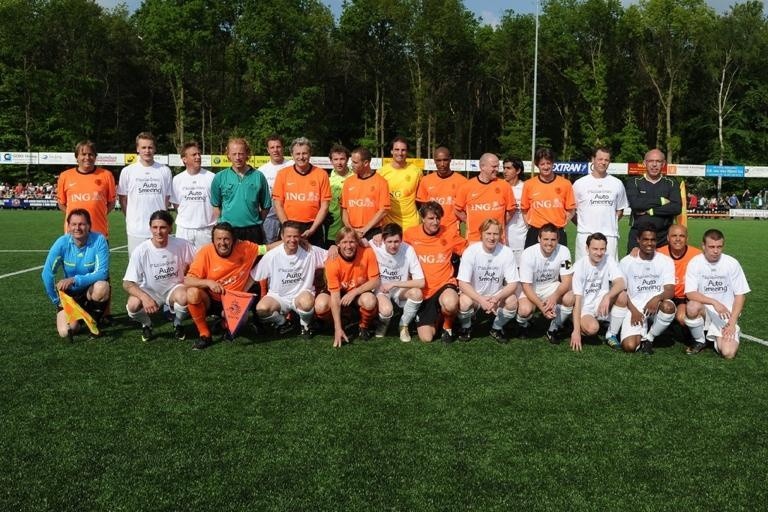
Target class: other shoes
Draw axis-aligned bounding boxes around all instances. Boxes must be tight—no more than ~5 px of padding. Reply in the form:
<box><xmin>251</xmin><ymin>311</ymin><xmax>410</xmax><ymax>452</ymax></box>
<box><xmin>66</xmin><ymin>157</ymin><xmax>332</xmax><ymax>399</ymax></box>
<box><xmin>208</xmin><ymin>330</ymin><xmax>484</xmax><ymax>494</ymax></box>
<box><xmin>98</xmin><ymin>313</ymin><xmax>118</xmax><ymax>327</ymax></box>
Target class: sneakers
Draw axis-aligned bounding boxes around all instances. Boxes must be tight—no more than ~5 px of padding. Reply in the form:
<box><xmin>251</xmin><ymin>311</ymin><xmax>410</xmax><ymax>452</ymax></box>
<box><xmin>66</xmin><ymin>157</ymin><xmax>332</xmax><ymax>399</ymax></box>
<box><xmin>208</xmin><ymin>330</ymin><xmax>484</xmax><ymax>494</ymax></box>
<box><xmin>358</xmin><ymin>324</ymin><xmax>369</xmax><ymax>340</ymax></box>
<box><xmin>141</xmin><ymin>323</ymin><xmax>152</xmax><ymax>341</ymax></box>
<box><xmin>640</xmin><ymin>338</ymin><xmax>654</xmax><ymax>354</ymax></box>
<box><xmin>488</xmin><ymin>327</ymin><xmax>508</xmax><ymax>344</ymax></box>
<box><xmin>458</xmin><ymin>326</ymin><xmax>472</xmax><ymax>341</ymax></box>
<box><xmin>686</xmin><ymin>341</ymin><xmax>707</xmax><ymax>354</ymax></box>
<box><xmin>271</xmin><ymin>319</ymin><xmax>294</xmax><ymax>339</ymax></box>
<box><xmin>441</xmin><ymin>327</ymin><xmax>455</xmax><ymax>342</ymax></box>
<box><xmin>196</xmin><ymin>336</ymin><xmax>214</xmax><ymax>349</ymax></box>
<box><xmin>173</xmin><ymin>321</ymin><xmax>185</xmax><ymax>340</ymax></box>
<box><xmin>301</xmin><ymin>321</ymin><xmax>313</xmax><ymax>336</ymax></box>
<box><xmin>375</xmin><ymin>324</ymin><xmax>387</xmax><ymax>338</ymax></box>
<box><xmin>545</xmin><ymin>329</ymin><xmax>559</xmax><ymax>347</ymax></box>
<box><xmin>399</xmin><ymin>325</ymin><xmax>411</xmax><ymax>342</ymax></box>
<box><xmin>606</xmin><ymin>335</ymin><xmax>621</xmax><ymax>349</ymax></box>
<box><xmin>517</xmin><ymin>324</ymin><xmax>529</xmax><ymax>340</ymax></box>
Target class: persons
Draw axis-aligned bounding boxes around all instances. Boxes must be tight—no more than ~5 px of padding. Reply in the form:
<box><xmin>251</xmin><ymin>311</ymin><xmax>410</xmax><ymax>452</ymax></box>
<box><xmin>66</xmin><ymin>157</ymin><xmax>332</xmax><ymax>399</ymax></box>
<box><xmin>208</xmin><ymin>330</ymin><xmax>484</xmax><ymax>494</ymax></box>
<box><xmin>328</xmin><ymin>137</ymin><xmax>528</xmax><ymax>276</ymax></box>
<box><xmin>56</xmin><ymin>139</ymin><xmax>116</xmax><ymax>322</ymax></box>
<box><xmin>328</xmin><ymin>222</ymin><xmax>426</xmax><ymax>342</ymax></box>
<box><xmin>521</xmin><ymin>147</ymin><xmax>683</xmax><ymax>260</ymax></box>
<box><xmin>118</xmin><ymin>135</ymin><xmax>331</xmax><ymax>321</ymax></box>
<box><xmin>619</xmin><ymin>224</ymin><xmax>703</xmax><ymax>354</ymax></box>
<box><xmin>123</xmin><ymin>210</ymin><xmax>198</xmax><ymax>342</ymax></box>
<box><xmin>314</xmin><ymin>227</ymin><xmax>381</xmax><ymax>348</ymax></box>
<box><xmin>41</xmin><ymin>207</ymin><xmax>111</xmax><ymax>338</ymax></box>
<box><xmin>685</xmin><ymin>229</ymin><xmax>752</xmax><ymax>359</ymax></box>
<box><xmin>568</xmin><ymin>232</ymin><xmax>628</xmax><ymax>350</ymax></box>
<box><xmin>184</xmin><ymin>222</ymin><xmax>308</xmax><ymax>349</ymax></box>
<box><xmin>688</xmin><ymin>189</ymin><xmax>762</xmax><ymax>220</ymax></box>
<box><xmin>0</xmin><ymin>180</ymin><xmax>59</xmax><ymax>210</ymax></box>
<box><xmin>374</xmin><ymin>201</ymin><xmax>469</xmax><ymax>342</ymax></box>
<box><xmin>244</xmin><ymin>220</ymin><xmax>369</xmax><ymax>339</ymax></box>
<box><xmin>456</xmin><ymin>218</ymin><xmax>519</xmax><ymax>344</ymax></box>
<box><xmin>517</xmin><ymin>223</ymin><xmax>576</xmax><ymax>345</ymax></box>
